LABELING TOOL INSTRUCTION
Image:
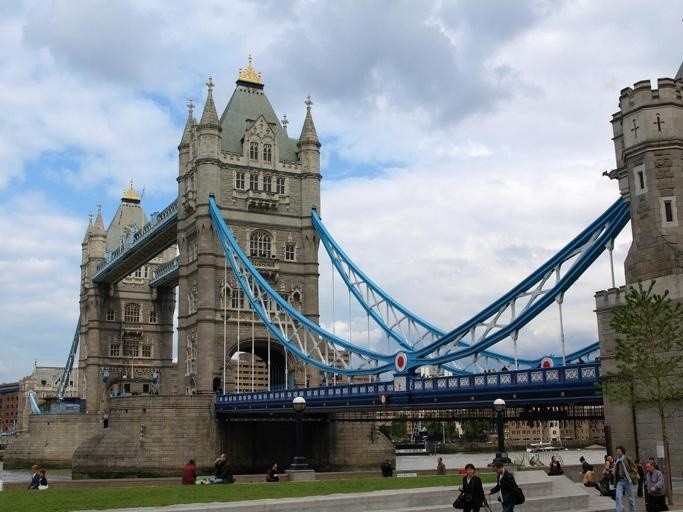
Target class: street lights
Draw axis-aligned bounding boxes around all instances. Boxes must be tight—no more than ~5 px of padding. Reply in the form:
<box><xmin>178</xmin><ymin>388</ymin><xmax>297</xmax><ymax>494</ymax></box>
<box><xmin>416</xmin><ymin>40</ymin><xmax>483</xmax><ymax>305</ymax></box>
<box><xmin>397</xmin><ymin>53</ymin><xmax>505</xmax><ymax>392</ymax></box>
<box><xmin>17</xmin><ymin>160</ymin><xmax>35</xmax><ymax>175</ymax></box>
<box><xmin>491</xmin><ymin>398</ymin><xmax>511</xmax><ymax>463</ymax></box>
<box><xmin>289</xmin><ymin>397</ymin><xmax>311</xmax><ymax>470</ymax></box>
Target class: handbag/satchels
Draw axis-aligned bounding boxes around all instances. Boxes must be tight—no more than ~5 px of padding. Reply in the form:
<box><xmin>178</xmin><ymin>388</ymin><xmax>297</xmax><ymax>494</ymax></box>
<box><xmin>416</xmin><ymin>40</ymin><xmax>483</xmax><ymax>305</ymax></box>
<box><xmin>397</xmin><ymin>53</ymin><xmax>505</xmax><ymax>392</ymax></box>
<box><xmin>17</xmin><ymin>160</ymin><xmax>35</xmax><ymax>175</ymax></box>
<box><xmin>453</xmin><ymin>490</ymin><xmax>473</xmax><ymax>509</ymax></box>
<box><xmin>507</xmin><ymin>487</ymin><xmax>525</xmax><ymax>504</ymax></box>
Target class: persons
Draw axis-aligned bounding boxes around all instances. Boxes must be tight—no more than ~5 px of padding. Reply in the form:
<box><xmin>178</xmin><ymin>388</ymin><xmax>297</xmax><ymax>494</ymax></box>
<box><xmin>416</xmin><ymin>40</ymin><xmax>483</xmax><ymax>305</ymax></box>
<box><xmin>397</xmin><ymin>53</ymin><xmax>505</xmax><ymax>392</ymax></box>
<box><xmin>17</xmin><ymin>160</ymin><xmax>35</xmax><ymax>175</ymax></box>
<box><xmin>380</xmin><ymin>458</ymin><xmax>393</xmax><ymax>477</ymax></box>
<box><xmin>213</xmin><ymin>451</ymin><xmax>234</xmax><ymax>483</ymax></box>
<box><xmin>483</xmin><ymin>356</ymin><xmax>598</xmax><ymax>374</ymax></box>
<box><xmin>435</xmin><ymin>457</ymin><xmax>446</xmax><ymax>474</ymax></box>
<box><xmin>598</xmin><ymin>471</ymin><xmax>616</xmax><ymax>500</ymax></box>
<box><xmin>582</xmin><ymin>464</ymin><xmax>601</xmax><ymax>492</ymax></box>
<box><xmin>483</xmin><ymin>460</ymin><xmax>517</xmax><ymax>511</ymax></box>
<box><xmin>27</xmin><ymin>464</ymin><xmax>40</xmax><ymax>489</ymax></box>
<box><xmin>578</xmin><ymin>455</ymin><xmax>590</xmax><ymax>474</ymax></box>
<box><xmin>605</xmin><ymin>454</ymin><xmax>616</xmax><ymax>485</ymax></box>
<box><xmin>612</xmin><ymin>445</ymin><xmax>639</xmax><ymax>511</ymax></box>
<box><xmin>634</xmin><ymin>458</ymin><xmax>645</xmax><ymax>496</ymax></box>
<box><xmin>648</xmin><ymin>456</ymin><xmax>660</xmax><ymax>471</ymax></box>
<box><xmin>181</xmin><ymin>459</ymin><xmax>196</xmax><ymax>485</ymax></box>
<box><xmin>458</xmin><ymin>463</ymin><xmax>488</xmax><ymax>512</ymax></box>
<box><xmin>35</xmin><ymin>468</ymin><xmax>48</xmax><ymax>490</ymax></box>
<box><xmin>265</xmin><ymin>462</ymin><xmax>279</xmax><ymax>481</ymax></box>
<box><xmin>549</xmin><ymin>455</ymin><xmax>562</xmax><ymax>475</ymax></box>
<box><xmin>643</xmin><ymin>461</ymin><xmax>666</xmax><ymax>511</ymax></box>
